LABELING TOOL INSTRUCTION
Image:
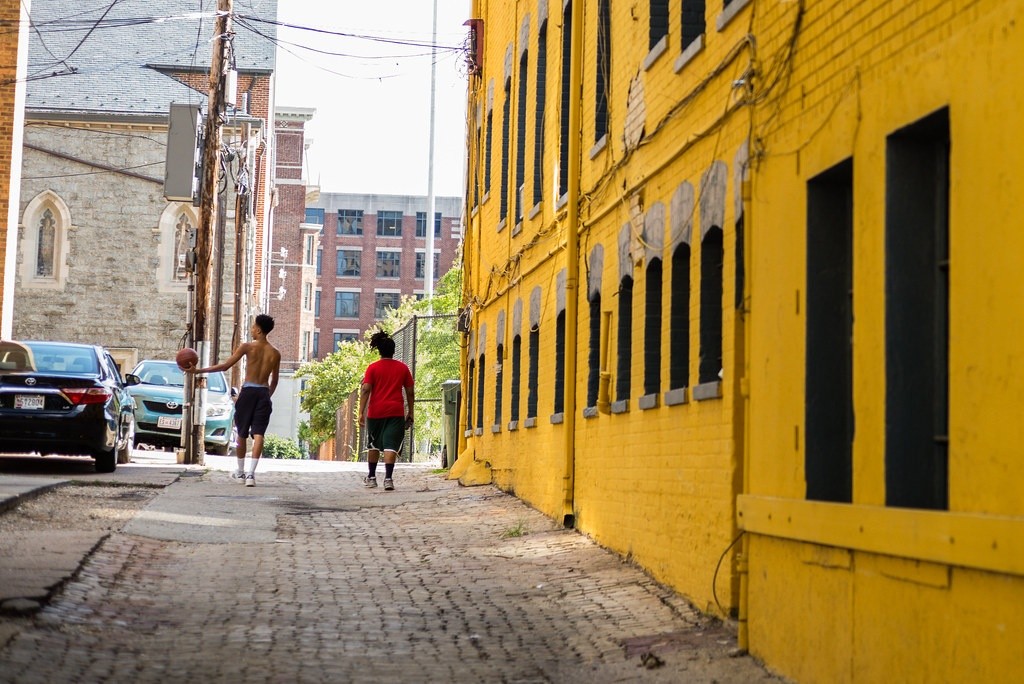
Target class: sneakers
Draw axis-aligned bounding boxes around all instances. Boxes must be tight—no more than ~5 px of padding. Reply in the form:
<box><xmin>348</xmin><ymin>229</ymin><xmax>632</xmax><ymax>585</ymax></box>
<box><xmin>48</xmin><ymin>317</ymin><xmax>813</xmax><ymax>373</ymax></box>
<box><xmin>383</xmin><ymin>478</ymin><xmax>394</xmax><ymax>490</ymax></box>
<box><xmin>246</xmin><ymin>475</ymin><xmax>255</xmax><ymax>486</ymax></box>
<box><xmin>362</xmin><ymin>477</ymin><xmax>377</xmax><ymax>487</ymax></box>
<box><xmin>231</xmin><ymin>473</ymin><xmax>245</xmax><ymax>484</ymax></box>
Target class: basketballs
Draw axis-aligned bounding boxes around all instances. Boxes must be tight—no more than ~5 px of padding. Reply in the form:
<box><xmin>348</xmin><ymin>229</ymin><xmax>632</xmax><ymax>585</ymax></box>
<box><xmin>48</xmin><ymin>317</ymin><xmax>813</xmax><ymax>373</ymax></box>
<box><xmin>176</xmin><ymin>348</ymin><xmax>199</xmax><ymax>369</ymax></box>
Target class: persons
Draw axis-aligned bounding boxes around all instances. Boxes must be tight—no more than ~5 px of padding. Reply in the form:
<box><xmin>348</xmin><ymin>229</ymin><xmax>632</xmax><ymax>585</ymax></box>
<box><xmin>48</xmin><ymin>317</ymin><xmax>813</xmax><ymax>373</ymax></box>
<box><xmin>179</xmin><ymin>314</ymin><xmax>281</xmax><ymax>486</ymax></box>
<box><xmin>359</xmin><ymin>338</ymin><xmax>414</xmax><ymax>489</ymax></box>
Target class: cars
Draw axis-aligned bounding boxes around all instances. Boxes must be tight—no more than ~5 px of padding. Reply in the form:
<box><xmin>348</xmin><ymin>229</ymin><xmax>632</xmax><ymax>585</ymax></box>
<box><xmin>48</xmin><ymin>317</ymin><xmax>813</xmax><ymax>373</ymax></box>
<box><xmin>126</xmin><ymin>358</ymin><xmax>238</xmax><ymax>458</ymax></box>
<box><xmin>0</xmin><ymin>341</ymin><xmax>142</xmax><ymax>474</ymax></box>
